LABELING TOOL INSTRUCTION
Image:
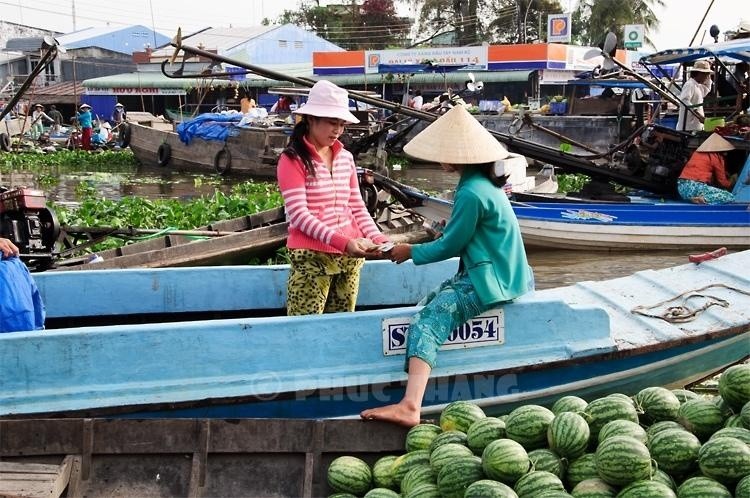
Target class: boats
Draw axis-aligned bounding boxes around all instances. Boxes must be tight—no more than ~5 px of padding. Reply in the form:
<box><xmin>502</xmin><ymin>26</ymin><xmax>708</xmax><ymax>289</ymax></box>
<box><xmin>0</xmin><ymin>247</ymin><xmax>750</xmax><ymax>414</ymax></box>
<box><xmin>1</xmin><ymin>117</ymin><xmax>119</xmax><ymax>156</ymax></box>
<box><xmin>0</xmin><ymin>165</ymin><xmax>445</xmax><ymax>267</ymax></box>
<box><xmin>0</xmin><ymin>413</ymin><xmax>439</xmax><ymax>498</ymax></box>
<box><xmin>152</xmin><ymin>29</ymin><xmax>750</xmax><ymax>249</ymax></box>
<box><xmin>116</xmin><ymin>96</ymin><xmax>440</xmax><ymax>181</ymax></box>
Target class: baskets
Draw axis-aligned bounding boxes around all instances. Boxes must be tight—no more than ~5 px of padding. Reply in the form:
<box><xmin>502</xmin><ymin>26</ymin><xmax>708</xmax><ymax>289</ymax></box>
<box><xmin>550</xmin><ymin>102</ymin><xmax>568</xmax><ymax>113</ymax></box>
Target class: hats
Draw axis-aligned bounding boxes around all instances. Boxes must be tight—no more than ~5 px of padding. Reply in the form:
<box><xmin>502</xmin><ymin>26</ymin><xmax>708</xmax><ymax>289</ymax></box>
<box><xmin>80</xmin><ymin>103</ymin><xmax>91</xmax><ymax>109</ymax></box>
<box><xmin>71</xmin><ymin>128</ymin><xmax>81</xmax><ymax>135</ymax></box>
<box><xmin>403</xmin><ymin>103</ymin><xmax>510</xmax><ymax>164</ymax></box>
<box><xmin>115</xmin><ymin>102</ymin><xmax>123</xmax><ymax>106</ymax></box>
<box><xmin>292</xmin><ymin>79</ymin><xmax>360</xmax><ymax>124</ymax></box>
<box><xmin>33</xmin><ymin>104</ymin><xmax>44</xmax><ymax>111</ymax></box>
<box><xmin>684</xmin><ymin>60</ymin><xmax>714</xmax><ymax>72</ymax></box>
<box><xmin>102</xmin><ymin>122</ymin><xmax>112</xmax><ymax>129</ymax></box>
<box><xmin>695</xmin><ymin>131</ymin><xmax>737</xmax><ymax>152</ymax></box>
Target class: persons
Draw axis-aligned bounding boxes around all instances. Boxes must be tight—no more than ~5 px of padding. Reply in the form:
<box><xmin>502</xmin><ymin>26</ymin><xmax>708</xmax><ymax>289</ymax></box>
<box><xmin>277</xmin><ymin>94</ymin><xmax>287</xmax><ymax>110</ymax></box>
<box><xmin>676</xmin><ymin>61</ymin><xmax>712</xmax><ymax>131</ymax></box>
<box><xmin>677</xmin><ymin>151</ymin><xmax>737</xmax><ymax>206</ymax></box>
<box><xmin>0</xmin><ymin>237</ymin><xmax>19</xmax><ymax>260</ymax></box>
<box><xmin>241</xmin><ymin>91</ymin><xmax>255</xmax><ymax>114</ymax></box>
<box><xmin>0</xmin><ymin>98</ymin><xmax>127</xmax><ymax>153</ymax></box>
<box><xmin>401</xmin><ymin>86</ymin><xmax>424</xmax><ymax>111</ymax></box>
<box><xmin>277</xmin><ymin>79</ymin><xmax>389</xmax><ymax>316</ymax></box>
<box><xmin>360</xmin><ymin>161</ymin><xmax>531</xmax><ymax>427</ymax></box>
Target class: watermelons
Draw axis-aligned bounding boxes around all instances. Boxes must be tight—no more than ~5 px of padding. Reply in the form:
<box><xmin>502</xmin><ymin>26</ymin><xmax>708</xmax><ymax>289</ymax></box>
<box><xmin>326</xmin><ymin>359</ymin><xmax>750</xmax><ymax>498</ymax></box>
